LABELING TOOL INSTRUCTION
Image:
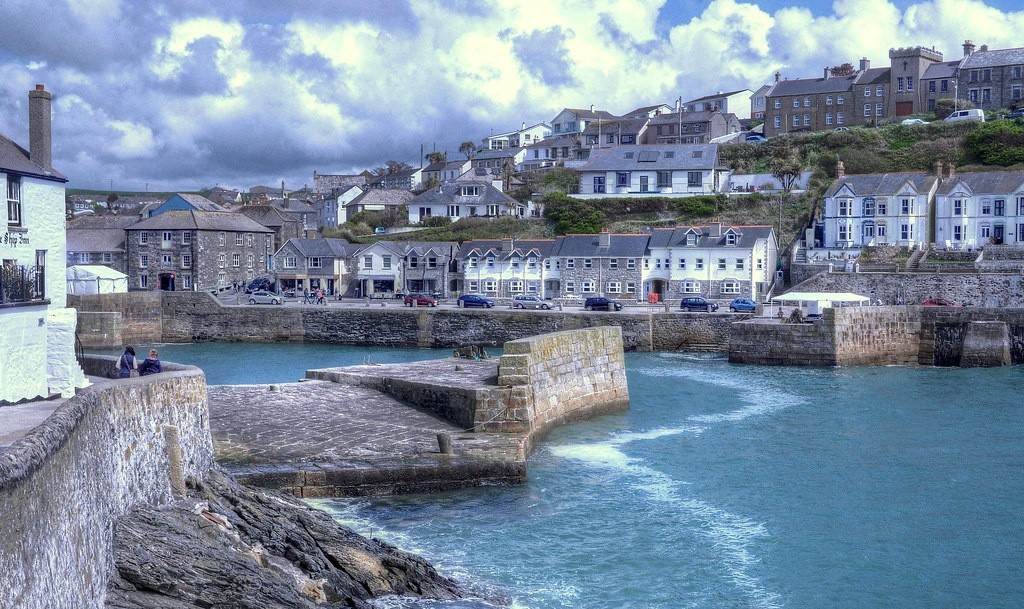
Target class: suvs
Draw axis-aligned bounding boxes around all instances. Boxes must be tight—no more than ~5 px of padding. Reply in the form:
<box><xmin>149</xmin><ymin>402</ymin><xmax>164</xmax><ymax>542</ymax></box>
<box><xmin>680</xmin><ymin>296</ymin><xmax>719</xmax><ymax>313</ymax></box>
<box><xmin>405</xmin><ymin>293</ymin><xmax>438</xmax><ymax>308</ymax></box>
<box><xmin>584</xmin><ymin>297</ymin><xmax>623</xmax><ymax>311</ymax></box>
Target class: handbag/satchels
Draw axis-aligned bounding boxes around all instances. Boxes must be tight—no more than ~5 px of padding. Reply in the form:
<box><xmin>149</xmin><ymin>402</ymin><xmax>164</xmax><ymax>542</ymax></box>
<box><xmin>130</xmin><ymin>370</ymin><xmax>139</xmax><ymax>378</ymax></box>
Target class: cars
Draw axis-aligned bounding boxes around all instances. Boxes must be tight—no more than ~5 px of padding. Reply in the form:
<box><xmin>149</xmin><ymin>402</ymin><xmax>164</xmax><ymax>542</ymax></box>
<box><xmin>730</xmin><ymin>298</ymin><xmax>760</xmax><ymax>313</ymax></box>
<box><xmin>923</xmin><ymin>298</ymin><xmax>963</xmax><ymax>308</ymax></box>
<box><xmin>745</xmin><ymin>135</ymin><xmax>768</xmax><ymax>143</ymax></box>
<box><xmin>246</xmin><ymin>278</ymin><xmax>271</xmax><ymax>294</ymax></box>
<box><xmin>248</xmin><ymin>290</ymin><xmax>286</xmax><ymax>305</ymax></box>
<box><xmin>902</xmin><ymin>120</ymin><xmax>930</xmax><ymax>126</ymax></box>
<box><xmin>456</xmin><ymin>295</ymin><xmax>492</xmax><ymax>308</ymax></box>
<box><xmin>1004</xmin><ymin>108</ymin><xmax>1024</xmax><ymax>119</ymax></box>
<box><xmin>512</xmin><ymin>295</ymin><xmax>555</xmax><ymax>310</ymax></box>
<box><xmin>833</xmin><ymin>127</ymin><xmax>849</xmax><ymax>132</ymax></box>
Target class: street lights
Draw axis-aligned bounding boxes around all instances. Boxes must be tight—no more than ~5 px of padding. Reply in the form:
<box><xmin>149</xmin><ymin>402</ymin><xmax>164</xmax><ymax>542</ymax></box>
<box><xmin>951</xmin><ymin>81</ymin><xmax>958</xmax><ymax>113</ymax></box>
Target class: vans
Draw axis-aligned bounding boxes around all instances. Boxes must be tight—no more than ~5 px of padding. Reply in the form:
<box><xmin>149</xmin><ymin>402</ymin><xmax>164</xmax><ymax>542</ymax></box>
<box><xmin>943</xmin><ymin>109</ymin><xmax>985</xmax><ymax>123</ymax></box>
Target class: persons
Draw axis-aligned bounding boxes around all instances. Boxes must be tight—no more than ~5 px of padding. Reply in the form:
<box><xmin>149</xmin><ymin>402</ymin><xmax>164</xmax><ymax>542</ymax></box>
<box><xmin>312</xmin><ymin>287</ymin><xmax>324</xmax><ymax>304</ymax></box>
<box><xmin>396</xmin><ymin>288</ymin><xmax>409</xmax><ymax>298</ymax></box>
<box><xmin>962</xmin><ymin>300</ymin><xmax>967</xmax><ymax>306</ymax></box>
<box><xmin>115</xmin><ymin>346</ymin><xmax>138</xmax><ymax>378</ymax></box>
<box><xmin>433</xmin><ymin>287</ymin><xmax>442</xmax><ymax>298</ymax></box>
<box><xmin>952</xmin><ymin>299</ymin><xmax>957</xmax><ymax>306</ymax></box>
<box><xmin>304</xmin><ymin>288</ymin><xmax>311</xmax><ymax>304</ymax></box>
<box><xmin>877</xmin><ymin>297</ymin><xmax>882</xmax><ymax>305</ymax></box>
<box><xmin>265</xmin><ymin>284</ymin><xmax>269</xmax><ymax>291</ymax></box>
<box><xmin>232</xmin><ymin>281</ymin><xmax>239</xmax><ymax>293</ymax></box>
<box><xmin>139</xmin><ymin>349</ymin><xmax>162</xmax><ymax>376</ymax></box>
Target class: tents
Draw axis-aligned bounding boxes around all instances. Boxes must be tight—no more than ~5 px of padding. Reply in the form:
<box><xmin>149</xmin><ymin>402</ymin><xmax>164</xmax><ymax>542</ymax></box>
<box><xmin>66</xmin><ymin>265</ymin><xmax>128</xmax><ymax>295</ymax></box>
